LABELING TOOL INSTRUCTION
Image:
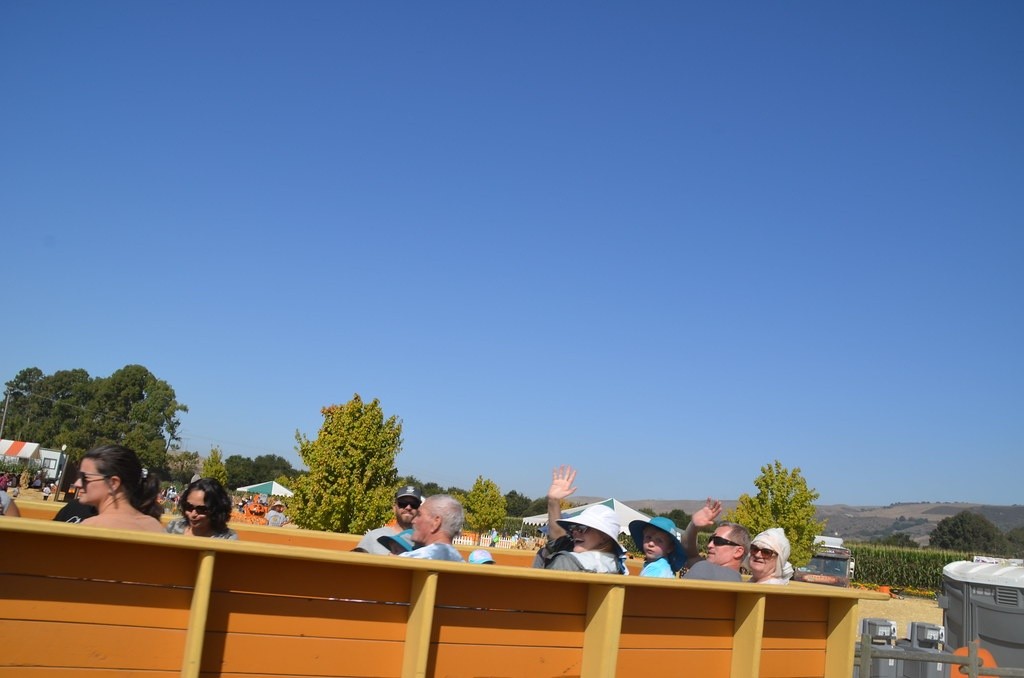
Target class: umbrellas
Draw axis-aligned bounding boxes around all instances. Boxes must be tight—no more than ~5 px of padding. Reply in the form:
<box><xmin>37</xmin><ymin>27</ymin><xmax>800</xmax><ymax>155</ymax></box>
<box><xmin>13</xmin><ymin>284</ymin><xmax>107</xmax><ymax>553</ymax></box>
<box><xmin>236</xmin><ymin>481</ymin><xmax>295</xmax><ymax>498</ymax></box>
<box><xmin>522</xmin><ymin>498</ymin><xmax>685</xmax><ymax>544</ymax></box>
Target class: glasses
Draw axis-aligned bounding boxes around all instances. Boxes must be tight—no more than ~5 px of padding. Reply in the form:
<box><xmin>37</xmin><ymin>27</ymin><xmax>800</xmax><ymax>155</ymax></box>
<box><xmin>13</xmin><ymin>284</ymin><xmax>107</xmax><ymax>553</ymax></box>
<box><xmin>568</xmin><ymin>524</ymin><xmax>591</xmax><ymax>534</ymax></box>
<box><xmin>397</xmin><ymin>499</ymin><xmax>420</xmax><ymax>509</ymax></box>
<box><xmin>79</xmin><ymin>471</ymin><xmax>111</xmax><ymax>487</ymax></box>
<box><xmin>749</xmin><ymin>544</ymin><xmax>779</xmax><ymax>558</ymax></box>
<box><xmin>708</xmin><ymin>535</ymin><xmax>741</xmax><ymax>548</ymax></box>
<box><xmin>185</xmin><ymin>502</ymin><xmax>208</xmax><ymax>514</ymax></box>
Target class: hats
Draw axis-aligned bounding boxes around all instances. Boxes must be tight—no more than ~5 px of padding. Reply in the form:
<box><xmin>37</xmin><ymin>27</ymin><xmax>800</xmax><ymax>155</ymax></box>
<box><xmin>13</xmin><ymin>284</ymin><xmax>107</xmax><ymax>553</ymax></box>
<box><xmin>468</xmin><ymin>550</ymin><xmax>497</xmax><ymax>564</ymax></box>
<box><xmin>271</xmin><ymin>501</ymin><xmax>285</xmax><ymax>512</ymax></box>
<box><xmin>629</xmin><ymin>517</ymin><xmax>686</xmax><ymax>572</ymax></box>
<box><xmin>537</xmin><ymin>513</ymin><xmax>570</xmax><ymax>535</ymax></box>
<box><xmin>351</xmin><ymin>528</ymin><xmax>395</xmax><ymax>555</ymax></box>
<box><xmin>397</xmin><ymin>486</ymin><xmax>423</xmax><ymax>503</ymax></box>
<box><xmin>556</xmin><ymin>504</ymin><xmax>627</xmax><ymax>554</ymax></box>
<box><xmin>377</xmin><ymin>528</ymin><xmax>421</xmax><ymax>553</ymax></box>
<box><xmin>750</xmin><ymin>527</ymin><xmax>790</xmax><ymax>567</ymax></box>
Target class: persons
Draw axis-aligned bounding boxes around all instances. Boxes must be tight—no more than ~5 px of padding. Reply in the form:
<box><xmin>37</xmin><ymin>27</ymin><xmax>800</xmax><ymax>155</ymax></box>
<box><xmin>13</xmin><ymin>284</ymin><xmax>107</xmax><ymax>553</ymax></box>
<box><xmin>397</xmin><ymin>494</ymin><xmax>466</xmax><ymax>563</ymax></box>
<box><xmin>532</xmin><ymin>465</ymin><xmax>630</xmax><ymax>576</ymax></box>
<box><xmin>468</xmin><ymin>549</ymin><xmax>497</xmax><ymax>565</ymax></box>
<box><xmin>348</xmin><ymin>484</ymin><xmax>426</xmax><ymax>561</ymax></box>
<box><xmin>488</xmin><ymin>527</ymin><xmax>496</xmax><ymax>547</ymax></box>
<box><xmin>0</xmin><ymin>471</ymin><xmax>20</xmax><ymax>517</ymax></box>
<box><xmin>31</xmin><ymin>477</ymin><xmax>51</xmax><ymax>501</ymax></box>
<box><xmin>513</xmin><ymin>528</ymin><xmax>525</xmax><ymax>551</ymax></box>
<box><xmin>166</xmin><ymin>477</ymin><xmax>238</xmax><ymax>540</ymax></box>
<box><xmin>628</xmin><ymin>516</ymin><xmax>687</xmax><ymax>579</ymax></box>
<box><xmin>52</xmin><ymin>444</ymin><xmax>181</xmax><ymax>534</ymax></box>
<box><xmin>679</xmin><ymin>495</ymin><xmax>750</xmax><ymax>582</ymax></box>
<box><xmin>748</xmin><ymin>528</ymin><xmax>795</xmax><ymax>586</ymax></box>
<box><xmin>236</xmin><ymin>495</ymin><xmax>291</xmax><ymax>526</ymax></box>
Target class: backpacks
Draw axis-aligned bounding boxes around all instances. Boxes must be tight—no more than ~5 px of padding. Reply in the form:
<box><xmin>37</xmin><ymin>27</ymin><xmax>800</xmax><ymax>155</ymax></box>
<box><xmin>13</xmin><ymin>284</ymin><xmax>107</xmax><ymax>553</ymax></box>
<box><xmin>493</xmin><ymin>535</ymin><xmax>500</xmax><ymax>543</ymax></box>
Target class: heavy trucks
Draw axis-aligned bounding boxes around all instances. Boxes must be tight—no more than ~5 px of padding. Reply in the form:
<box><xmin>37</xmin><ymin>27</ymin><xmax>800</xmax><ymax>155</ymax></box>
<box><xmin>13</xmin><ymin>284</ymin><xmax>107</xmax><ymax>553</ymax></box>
<box><xmin>792</xmin><ymin>536</ymin><xmax>855</xmax><ymax>587</ymax></box>
